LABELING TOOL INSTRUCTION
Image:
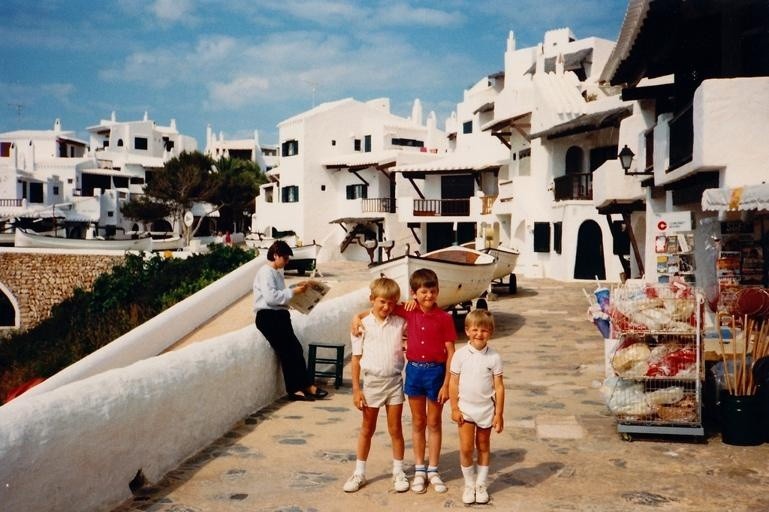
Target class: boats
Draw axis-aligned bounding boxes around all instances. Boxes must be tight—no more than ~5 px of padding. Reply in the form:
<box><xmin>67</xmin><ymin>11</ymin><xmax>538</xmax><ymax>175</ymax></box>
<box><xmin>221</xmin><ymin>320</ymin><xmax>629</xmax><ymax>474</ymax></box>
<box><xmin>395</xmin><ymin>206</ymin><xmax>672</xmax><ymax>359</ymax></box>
<box><xmin>15</xmin><ymin>228</ymin><xmax>152</xmax><ymax>251</ymax></box>
<box><xmin>140</xmin><ymin>232</ymin><xmax>184</xmax><ymax>252</ymax></box>
<box><xmin>358</xmin><ymin>236</ymin><xmax>495</xmax><ymax>316</ymax></box>
<box><xmin>285</xmin><ymin>240</ymin><xmax>322</xmax><ymax>275</ymax></box>
<box><xmin>458</xmin><ymin>222</ymin><xmax>519</xmax><ymax>293</ymax></box>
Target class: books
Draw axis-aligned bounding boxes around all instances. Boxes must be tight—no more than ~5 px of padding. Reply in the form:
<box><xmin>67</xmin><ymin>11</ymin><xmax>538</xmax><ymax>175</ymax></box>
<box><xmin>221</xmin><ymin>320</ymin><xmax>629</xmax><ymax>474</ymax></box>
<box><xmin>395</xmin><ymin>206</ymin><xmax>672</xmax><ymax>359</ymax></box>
<box><xmin>656</xmin><ymin>231</ymin><xmax>698</xmax><ymax>286</ymax></box>
<box><xmin>714</xmin><ymin>232</ymin><xmax>769</xmax><ymax>346</ymax></box>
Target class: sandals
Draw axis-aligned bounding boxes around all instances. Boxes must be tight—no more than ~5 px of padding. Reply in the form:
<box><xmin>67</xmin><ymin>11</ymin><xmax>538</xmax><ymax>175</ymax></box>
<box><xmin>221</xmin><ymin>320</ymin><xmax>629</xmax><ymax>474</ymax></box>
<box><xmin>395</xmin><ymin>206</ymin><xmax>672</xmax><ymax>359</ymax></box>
<box><xmin>429</xmin><ymin>474</ymin><xmax>447</xmax><ymax>492</ymax></box>
<box><xmin>411</xmin><ymin>474</ymin><xmax>428</xmax><ymax>493</ymax></box>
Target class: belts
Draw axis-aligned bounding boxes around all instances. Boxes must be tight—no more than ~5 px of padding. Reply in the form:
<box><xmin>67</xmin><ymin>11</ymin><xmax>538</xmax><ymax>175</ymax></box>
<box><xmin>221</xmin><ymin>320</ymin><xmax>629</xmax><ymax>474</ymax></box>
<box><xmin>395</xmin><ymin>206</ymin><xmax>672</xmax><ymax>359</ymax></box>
<box><xmin>410</xmin><ymin>362</ymin><xmax>439</xmax><ymax>368</ymax></box>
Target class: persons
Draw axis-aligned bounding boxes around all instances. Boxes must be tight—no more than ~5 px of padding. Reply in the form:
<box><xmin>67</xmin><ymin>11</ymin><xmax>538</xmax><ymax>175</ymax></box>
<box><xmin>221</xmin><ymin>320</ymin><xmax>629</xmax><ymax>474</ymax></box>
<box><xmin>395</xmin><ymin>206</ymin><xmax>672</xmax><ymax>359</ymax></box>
<box><xmin>214</xmin><ymin>232</ymin><xmax>224</xmax><ymax>244</ymax></box>
<box><xmin>223</xmin><ymin>230</ymin><xmax>233</xmax><ymax>245</ymax></box>
<box><xmin>342</xmin><ymin>278</ymin><xmax>417</xmax><ymax>494</ymax></box>
<box><xmin>350</xmin><ymin>268</ymin><xmax>456</xmax><ymax>495</ymax></box>
<box><xmin>449</xmin><ymin>309</ymin><xmax>504</xmax><ymax>505</ymax></box>
<box><xmin>253</xmin><ymin>241</ymin><xmax>329</xmax><ymax>401</ymax></box>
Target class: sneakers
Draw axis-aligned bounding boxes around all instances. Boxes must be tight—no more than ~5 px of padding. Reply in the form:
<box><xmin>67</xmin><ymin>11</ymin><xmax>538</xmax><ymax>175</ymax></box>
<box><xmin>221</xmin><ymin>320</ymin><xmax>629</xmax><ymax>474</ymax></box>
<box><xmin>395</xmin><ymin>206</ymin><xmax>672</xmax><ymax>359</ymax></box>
<box><xmin>393</xmin><ymin>471</ymin><xmax>410</xmax><ymax>492</ymax></box>
<box><xmin>474</xmin><ymin>484</ymin><xmax>489</xmax><ymax>504</ymax></box>
<box><xmin>343</xmin><ymin>474</ymin><xmax>367</xmax><ymax>491</ymax></box>
<box><xmin>462</xmin><ymin>484</ymin><xmax>475</xmax><ymax>504</ymax></box>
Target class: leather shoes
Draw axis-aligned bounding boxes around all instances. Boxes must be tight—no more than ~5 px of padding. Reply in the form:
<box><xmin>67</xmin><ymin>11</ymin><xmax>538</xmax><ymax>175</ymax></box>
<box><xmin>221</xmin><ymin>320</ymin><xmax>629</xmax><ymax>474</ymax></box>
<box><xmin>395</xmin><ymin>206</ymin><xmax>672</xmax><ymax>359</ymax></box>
<box><xmin>289</xmin><ymin>394</ymin><xmax>313</xmax><ymax>400</ymax></box>
<box><xmin>310</xmin><ymin>387</ymin><xmax>328</xmax><ymax>398</ymax></box>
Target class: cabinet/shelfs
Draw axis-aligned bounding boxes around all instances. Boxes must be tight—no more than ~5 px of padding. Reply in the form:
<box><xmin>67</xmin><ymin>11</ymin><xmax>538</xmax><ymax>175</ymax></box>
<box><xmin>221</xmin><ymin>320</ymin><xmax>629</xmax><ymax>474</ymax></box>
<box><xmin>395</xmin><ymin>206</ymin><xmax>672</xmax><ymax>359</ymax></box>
<box><xmin>740</xmin><ymin>245</ymin><xmax>769</xmax><ymax>286</ymax></box>
<box><xmin>613</xmin><ymin>279</ymin><xmax>706</xmax><ymax>442</ymax></box>
<box><xmin>718</xmin><ymin>249</ymin><xmax>740</xmax><ymax>295</ymax></box>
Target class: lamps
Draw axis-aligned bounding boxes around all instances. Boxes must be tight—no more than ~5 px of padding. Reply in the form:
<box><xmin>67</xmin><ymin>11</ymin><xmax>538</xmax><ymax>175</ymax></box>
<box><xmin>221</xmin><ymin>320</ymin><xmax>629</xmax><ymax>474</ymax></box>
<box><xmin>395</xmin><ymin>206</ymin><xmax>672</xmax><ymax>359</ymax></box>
<box><xmin>619</xmin><ymin>145</ymin><xmax>654</xmax><ymax>176</ymax></box>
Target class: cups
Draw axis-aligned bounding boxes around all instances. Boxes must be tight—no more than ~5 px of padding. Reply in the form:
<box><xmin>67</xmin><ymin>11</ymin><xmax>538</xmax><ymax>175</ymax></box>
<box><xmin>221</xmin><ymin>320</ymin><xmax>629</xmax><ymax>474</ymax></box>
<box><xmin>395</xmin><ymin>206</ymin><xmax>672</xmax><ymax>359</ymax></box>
<box><xmin>590</xmin><ymin>314</ymin><xmax>609</xmax><ymax>338</ymax></box>
<box><xmin>593</xmin><ymin>289</ymin><xmax>610</xmax><ymax>313</ymax></box>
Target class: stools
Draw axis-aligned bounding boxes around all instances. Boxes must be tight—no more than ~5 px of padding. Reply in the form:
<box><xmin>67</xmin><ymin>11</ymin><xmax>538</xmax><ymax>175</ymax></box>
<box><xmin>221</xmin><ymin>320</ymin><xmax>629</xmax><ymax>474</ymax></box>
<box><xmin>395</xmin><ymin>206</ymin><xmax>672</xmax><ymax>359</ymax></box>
<box><xmin>307</xmin><ymin>338</ymin><xmax>346</xmax><ymax>390</ymax></box>
